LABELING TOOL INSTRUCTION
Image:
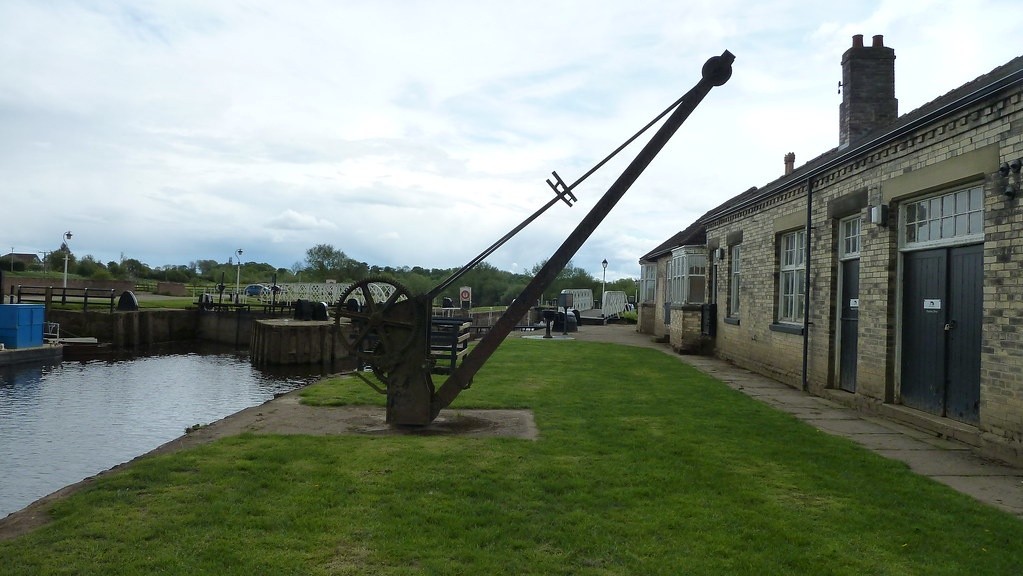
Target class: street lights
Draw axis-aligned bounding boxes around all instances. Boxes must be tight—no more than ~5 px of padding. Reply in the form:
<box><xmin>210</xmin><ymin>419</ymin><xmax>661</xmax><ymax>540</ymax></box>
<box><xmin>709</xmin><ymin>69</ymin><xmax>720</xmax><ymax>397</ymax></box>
<box><xmin>61</xmin><ymin>231</ymin><xmax>73</xmax><ymax>304</ymax></box>
<box><xmin>234</xmin><ymin>248</ymin><xmax>244</xmax><ymax>303</ymax></box>
<box><xmin>602</xmin><ymin>259</ymin><xmax>608</xmax><ymax>293</ymax></box>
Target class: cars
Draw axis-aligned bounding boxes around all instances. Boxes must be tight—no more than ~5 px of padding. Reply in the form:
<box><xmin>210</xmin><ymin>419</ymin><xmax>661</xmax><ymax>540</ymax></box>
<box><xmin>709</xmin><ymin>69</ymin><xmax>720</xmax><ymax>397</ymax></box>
<box><xmin>628</xmin><ymin>303</ymin><xmax>635</xmax><ymax>309</ymax></box>
<box><xmin>243</xmin><ymin>283</ymin><xmax>272</xmax><ymax>297</ymax></box>
<box><xmin>265</xmin><ymin>284</ymin><xmax>287</xmax><ymax>294</ymax></box>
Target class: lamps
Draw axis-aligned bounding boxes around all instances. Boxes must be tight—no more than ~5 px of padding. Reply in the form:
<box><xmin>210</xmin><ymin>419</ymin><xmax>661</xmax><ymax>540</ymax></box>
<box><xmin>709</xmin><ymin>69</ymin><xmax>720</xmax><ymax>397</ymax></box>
<box><xmin>875</xmin><ymin>204</ymin><xmax>889</xmax><ymax>228</ymax></box>
<box><xmin>719</xmin><ymin>248</ymin><xmax>727</xmax><ymax>262</ymax></box>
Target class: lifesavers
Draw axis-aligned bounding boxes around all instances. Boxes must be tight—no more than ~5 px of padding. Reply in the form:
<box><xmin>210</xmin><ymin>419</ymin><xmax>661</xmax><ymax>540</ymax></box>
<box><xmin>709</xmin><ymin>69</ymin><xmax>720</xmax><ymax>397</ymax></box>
<box><xmin>198</xmin><ymin>293</ymin><xmax>213</xmax><ymax>310</ymax></box>
